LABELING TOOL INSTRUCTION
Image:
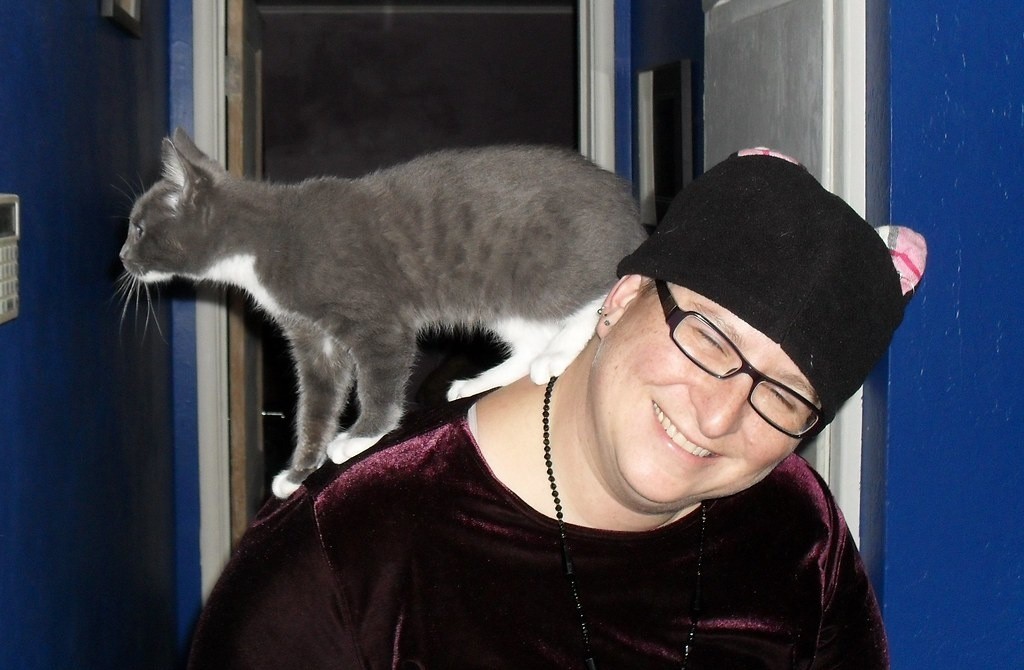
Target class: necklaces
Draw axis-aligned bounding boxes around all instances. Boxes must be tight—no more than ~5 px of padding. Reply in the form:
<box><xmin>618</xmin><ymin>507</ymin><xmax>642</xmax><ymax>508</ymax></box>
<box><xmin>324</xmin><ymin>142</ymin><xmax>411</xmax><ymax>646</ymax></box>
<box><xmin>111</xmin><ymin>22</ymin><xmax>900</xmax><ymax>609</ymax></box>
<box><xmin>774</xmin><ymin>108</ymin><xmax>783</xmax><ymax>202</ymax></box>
<box><xmin>542</xmin><ymin>375</ymin><xmax>707</xmax><ymax>670</ymax></box>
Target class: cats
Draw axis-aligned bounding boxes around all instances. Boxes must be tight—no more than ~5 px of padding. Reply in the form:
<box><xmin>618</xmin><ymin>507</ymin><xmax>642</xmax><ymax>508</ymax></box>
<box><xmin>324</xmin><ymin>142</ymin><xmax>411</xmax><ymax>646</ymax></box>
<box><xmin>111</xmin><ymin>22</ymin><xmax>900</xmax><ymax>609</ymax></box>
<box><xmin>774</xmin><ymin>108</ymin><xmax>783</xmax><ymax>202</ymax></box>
<box><xmin>104</xmin><ymin>127</ymin><xmax>649</xmax><ymax>501</ymax></box>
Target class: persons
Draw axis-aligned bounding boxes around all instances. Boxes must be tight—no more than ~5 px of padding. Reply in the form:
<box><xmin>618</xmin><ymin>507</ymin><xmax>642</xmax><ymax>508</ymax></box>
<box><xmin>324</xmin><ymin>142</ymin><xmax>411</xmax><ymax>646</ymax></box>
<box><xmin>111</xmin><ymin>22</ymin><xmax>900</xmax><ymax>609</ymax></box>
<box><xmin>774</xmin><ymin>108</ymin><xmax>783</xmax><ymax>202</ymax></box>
<box><xmin>178</xmin><ymin>146</ymin><xmax>929</xmax><ymax>670</ymax></box>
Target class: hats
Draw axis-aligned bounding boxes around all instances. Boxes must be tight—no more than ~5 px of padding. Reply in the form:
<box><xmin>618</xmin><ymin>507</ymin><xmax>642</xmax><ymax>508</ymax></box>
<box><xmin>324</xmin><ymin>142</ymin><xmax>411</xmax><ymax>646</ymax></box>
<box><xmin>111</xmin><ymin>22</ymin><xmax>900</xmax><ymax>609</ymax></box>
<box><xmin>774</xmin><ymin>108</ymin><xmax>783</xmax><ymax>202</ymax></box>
<box><xmin>615</xmin><ymin>146</ymin><xmax>927</xmax><ymax>426</ymax></box>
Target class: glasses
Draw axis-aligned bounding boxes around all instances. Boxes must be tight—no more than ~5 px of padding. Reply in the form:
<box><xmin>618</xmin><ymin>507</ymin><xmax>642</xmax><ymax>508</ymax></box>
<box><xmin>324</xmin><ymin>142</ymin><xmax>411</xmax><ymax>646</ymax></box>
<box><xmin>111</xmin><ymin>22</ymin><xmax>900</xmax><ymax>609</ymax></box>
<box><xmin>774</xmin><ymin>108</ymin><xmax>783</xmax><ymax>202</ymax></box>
<box><xmin>656</xmin><ymin>278</ymin><xmax>821</xmax><ymax>440</ymax></box>
